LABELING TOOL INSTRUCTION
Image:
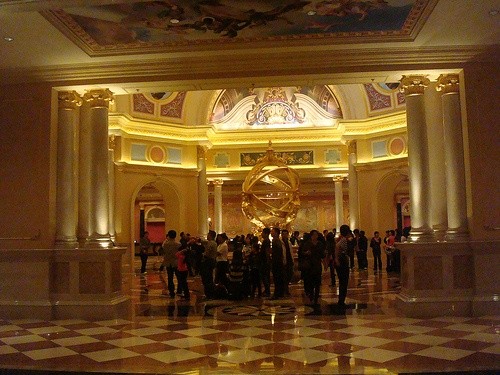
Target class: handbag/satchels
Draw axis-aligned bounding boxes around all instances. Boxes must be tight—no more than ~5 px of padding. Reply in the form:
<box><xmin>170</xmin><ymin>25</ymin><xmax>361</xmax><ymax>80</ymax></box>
<box><xmin>297</xmin><ymin>260</ymin><xmax>310</xmax><ymax>270</ymax></box>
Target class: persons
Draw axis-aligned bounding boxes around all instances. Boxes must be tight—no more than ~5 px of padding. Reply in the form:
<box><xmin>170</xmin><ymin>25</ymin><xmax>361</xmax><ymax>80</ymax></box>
<box><xmin>158</xmin><ymin>231</ymin><xmax>205</xmax><ymax>278</ymax></box>
<box><xmin>323</xmin><ymin>228</ymin><xmax>336</xmax><ymax>288</ymax></box>
<box><xmin>269</xmin><ymin>227</ymin><xmax>283</xmax><ymax>301</ymax></box>
<box><xmin>347</xmin><ymin>228</ymin><xmax>368</xmax><ymax>271</ymax></box>
<box><xmin>139</xmin><ymin>231</ymin><xmax>150</xmax><ymax>275</ymax></box>
<box><xmin>280</xmin><ymin>229</ymin><xmax>295</xmax><ymax>296</ymax></box>
<box><xmin>383</xmin><ymin>227</ymin><xmax>402</xmax><ymax>273</ymax></box>
<box><xmin>300</xmin><ymin>230</ymin><xmax>324</xmax><ymax>304</ymax></box>
<box><xmin>175</xmin><ymin>249</ymin><xmax>190</xmax><ymax>302</ymax></box>
<box><xmin>335</xmin><ymin>225</ymin><xmax>351</xmax><ymax>309</ymax></box>
<box><xmin>215</xmin><ymin>234</ymin><xmax>233</xmax><ymax>297</ymax></box>
<box><xmin>163</xmin><ymin>229</ymin><xmax>183</xmax><ymax>296</ymax></box>
<box><xmin>233</xmin><ymin>233</ymin><xmax>261</xmax><ymax>299</ymax></box>
<box><xmin>369</xmin><ymin>231</ymin><xmax>382</xmax><ymax>270</ymax></box>
<box><xmin>197</xmin><ymin>230</ymin><xmax>218</xmax><ymax>299</ymax></box>
<box><xmin>290</xmin><ymin>231</ymin><xmax>300</xmax><ymax>245</ymax></box>
<box><xmin>260</xmin><ymin>227</ymin><xmax>271</xmax><ymax>298</ymax></box>
